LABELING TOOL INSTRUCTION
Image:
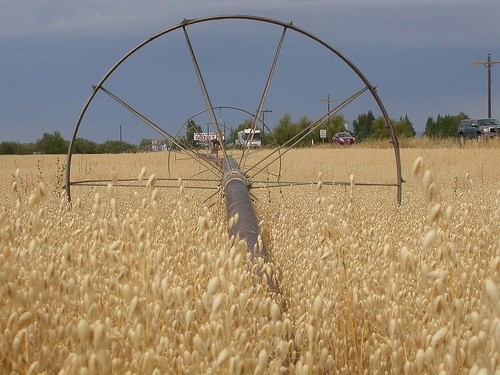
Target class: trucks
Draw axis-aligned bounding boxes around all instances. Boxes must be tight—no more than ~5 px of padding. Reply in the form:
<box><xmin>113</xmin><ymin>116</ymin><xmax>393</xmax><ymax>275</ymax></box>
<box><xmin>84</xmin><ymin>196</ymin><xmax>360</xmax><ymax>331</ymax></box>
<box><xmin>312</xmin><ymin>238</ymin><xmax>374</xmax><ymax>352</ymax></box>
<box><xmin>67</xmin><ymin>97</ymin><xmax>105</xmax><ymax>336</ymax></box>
<box><xmin>234</xmin><ymin>129</ymin><xmax>262</xmax><ymax>149</ymax></box>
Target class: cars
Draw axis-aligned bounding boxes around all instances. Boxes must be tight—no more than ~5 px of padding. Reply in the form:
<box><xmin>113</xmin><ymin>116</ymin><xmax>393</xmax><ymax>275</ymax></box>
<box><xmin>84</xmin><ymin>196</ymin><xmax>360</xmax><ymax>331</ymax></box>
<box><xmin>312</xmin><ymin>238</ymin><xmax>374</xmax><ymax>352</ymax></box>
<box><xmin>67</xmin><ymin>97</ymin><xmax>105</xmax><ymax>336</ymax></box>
<box><xmin>332</xmin><ymin>132</ymin><xmax>355</xmax><ymax>145</ymax></box>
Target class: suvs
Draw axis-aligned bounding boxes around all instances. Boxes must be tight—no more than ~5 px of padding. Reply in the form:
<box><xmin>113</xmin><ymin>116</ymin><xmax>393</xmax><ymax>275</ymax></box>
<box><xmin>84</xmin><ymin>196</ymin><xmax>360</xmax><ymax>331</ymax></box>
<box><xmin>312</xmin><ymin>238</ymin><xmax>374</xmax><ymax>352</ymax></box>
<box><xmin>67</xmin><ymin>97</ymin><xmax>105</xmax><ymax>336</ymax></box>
<box><xmin>457</xmin><ymin>119</ymin><xmax>500</xmax><ymax>139</ymax></box>
